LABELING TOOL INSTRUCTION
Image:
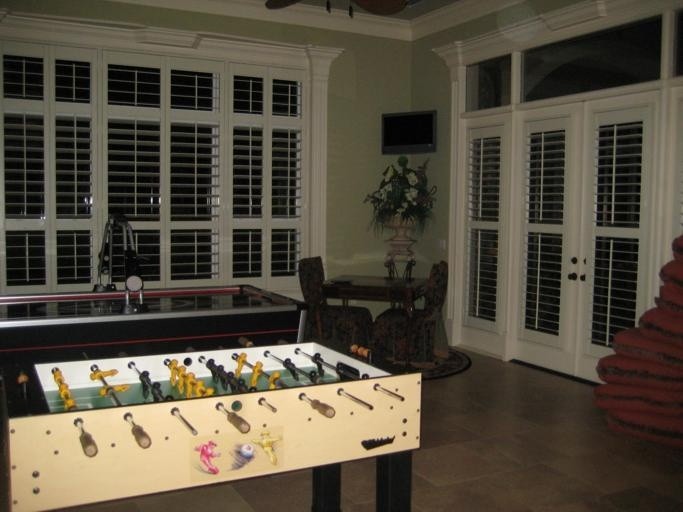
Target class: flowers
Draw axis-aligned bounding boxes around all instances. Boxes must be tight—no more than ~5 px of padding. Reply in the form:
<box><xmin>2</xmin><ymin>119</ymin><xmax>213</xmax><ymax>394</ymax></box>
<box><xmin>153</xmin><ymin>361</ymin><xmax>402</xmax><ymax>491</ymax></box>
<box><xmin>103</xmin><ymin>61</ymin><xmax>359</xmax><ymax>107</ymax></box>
<box><xmin>363</xmin><ymin>156</ymin><xmax>437</xmax><ymax>237</ymax></box>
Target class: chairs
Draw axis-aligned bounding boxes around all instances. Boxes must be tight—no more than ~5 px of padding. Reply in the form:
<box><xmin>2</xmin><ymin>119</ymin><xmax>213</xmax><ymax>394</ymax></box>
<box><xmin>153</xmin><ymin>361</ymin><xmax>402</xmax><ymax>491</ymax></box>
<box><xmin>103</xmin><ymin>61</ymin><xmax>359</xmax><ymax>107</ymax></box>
<box><xmin>375</xmin><ymin>261</ymin><xmax>449</xmax><ymax>361</ymax></box>
<box><xmin>300</xmin><ymin>255</ymin><xmax>374</xmax><ymax>358</ymax></box>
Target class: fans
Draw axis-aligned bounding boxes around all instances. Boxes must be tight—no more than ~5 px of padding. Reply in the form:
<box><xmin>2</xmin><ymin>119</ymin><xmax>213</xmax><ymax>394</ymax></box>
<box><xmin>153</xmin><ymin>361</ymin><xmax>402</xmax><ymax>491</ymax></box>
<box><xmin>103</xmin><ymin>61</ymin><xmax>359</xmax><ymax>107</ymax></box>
<box><xmin>265</xmin><ymin>0</ymin><xmax>303</xmax><ymax>10</ymax></box>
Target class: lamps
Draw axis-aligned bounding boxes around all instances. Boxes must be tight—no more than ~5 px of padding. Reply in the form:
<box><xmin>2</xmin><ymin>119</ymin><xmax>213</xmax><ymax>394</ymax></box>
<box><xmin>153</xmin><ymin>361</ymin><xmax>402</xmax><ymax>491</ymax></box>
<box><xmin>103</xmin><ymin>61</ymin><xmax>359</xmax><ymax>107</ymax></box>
<box><xmin>326</xmin><ymin>0</ymin><xmax>410</xmax><ymax>18</ymax></box>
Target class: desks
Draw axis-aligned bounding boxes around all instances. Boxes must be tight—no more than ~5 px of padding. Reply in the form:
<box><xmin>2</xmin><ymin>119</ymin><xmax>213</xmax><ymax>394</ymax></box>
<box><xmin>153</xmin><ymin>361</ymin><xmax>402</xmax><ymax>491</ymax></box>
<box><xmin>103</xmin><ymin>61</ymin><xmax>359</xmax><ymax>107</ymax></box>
<box><xmin>0</xmin><ymin>283</ymin><xmax>308</xmax><ymax>363</ymax></box>
<box><xmin>323</xmin><ymin>273</ymin><xmax>427</xmax><ymax>308</ymax></box>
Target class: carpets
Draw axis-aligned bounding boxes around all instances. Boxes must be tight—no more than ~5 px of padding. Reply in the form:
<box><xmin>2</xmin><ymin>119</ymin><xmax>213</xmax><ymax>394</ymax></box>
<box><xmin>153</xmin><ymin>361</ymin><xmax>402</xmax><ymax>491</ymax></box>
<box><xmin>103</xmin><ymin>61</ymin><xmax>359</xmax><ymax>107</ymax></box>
<box><xmin>371</xmin><ymin>347</ymin><xmax>471</xmax><ymax>380</ymax></box>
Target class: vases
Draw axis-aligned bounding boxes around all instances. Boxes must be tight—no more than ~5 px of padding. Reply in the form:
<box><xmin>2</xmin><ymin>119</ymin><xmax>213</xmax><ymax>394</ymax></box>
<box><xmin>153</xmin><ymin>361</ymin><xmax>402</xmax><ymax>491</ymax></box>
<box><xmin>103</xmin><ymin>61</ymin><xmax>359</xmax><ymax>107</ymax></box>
<box><xmin>382</xmin><ymin>210</ymin><xmax>424</xmax><ymax>280</ymax></box>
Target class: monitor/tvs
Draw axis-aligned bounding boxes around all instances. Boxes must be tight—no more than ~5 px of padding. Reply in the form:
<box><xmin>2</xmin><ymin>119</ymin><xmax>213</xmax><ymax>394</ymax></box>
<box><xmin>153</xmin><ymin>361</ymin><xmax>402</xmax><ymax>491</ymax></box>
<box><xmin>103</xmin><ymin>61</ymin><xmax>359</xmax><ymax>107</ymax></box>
<box><xmin>381</xmin><ymin>110</ymin><xmax>437</xmax><ymax>155</ymax></box>
